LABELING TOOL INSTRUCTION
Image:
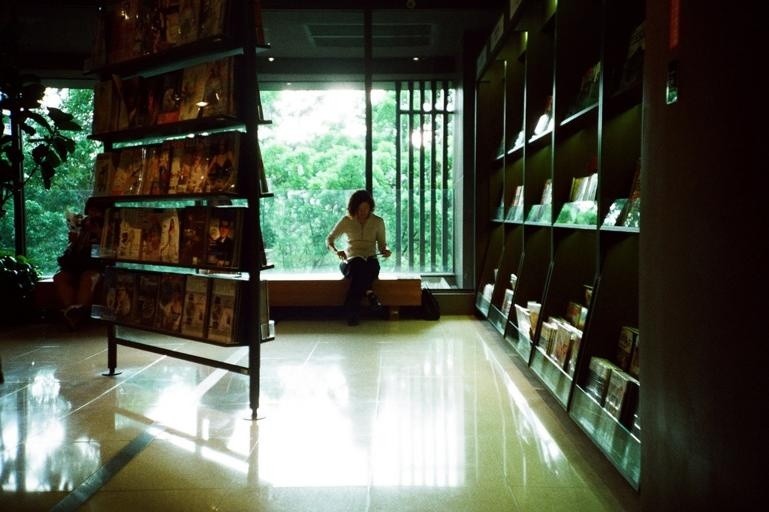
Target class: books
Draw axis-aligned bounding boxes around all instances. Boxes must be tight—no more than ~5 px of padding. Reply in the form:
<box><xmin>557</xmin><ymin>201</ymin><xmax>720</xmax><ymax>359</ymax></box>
<box><xmin>478</xmin><ymin>262</ymin><xmax>643</xmax><ymax>444</ymax></box>
<box><xmin>488</xmin><ymin>173</ymin><xmax>643</xmax><ymax>229</ymax></box>
<box><xmin>92</xmin><ymin>0</ymin><xmax>236</xmax><ymax>133</ymax></box>
<box><xmin>88</xmin><ymin>131</ymin><xmax>244</xmax><ymax>345</ymax></box>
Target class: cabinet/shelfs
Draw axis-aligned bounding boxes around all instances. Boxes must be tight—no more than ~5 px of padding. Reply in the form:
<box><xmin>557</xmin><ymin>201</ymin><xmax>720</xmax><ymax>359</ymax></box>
<box><xmin>472</xmin><ymin>2</ymin><xmax>642</xmax><ymax>486</ymax></box>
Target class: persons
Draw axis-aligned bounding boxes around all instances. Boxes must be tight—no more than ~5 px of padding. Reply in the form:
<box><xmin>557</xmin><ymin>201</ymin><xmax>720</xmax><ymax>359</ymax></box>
<box><xmin>53</xmin><ymin>194</ymin><xmax>114</xmax><ymax>323</ymax></box>
<box><xmin>324</xmin><ymin>192</ymin><xmax>391</xmax><ymax>326</ymax></box>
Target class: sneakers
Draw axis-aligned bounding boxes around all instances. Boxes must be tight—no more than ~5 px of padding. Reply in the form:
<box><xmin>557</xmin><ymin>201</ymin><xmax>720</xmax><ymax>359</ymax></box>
<box><xmin>368</xmin><ymin>293</ymin><xmax>382</xmax><ymax>309</ymax></box>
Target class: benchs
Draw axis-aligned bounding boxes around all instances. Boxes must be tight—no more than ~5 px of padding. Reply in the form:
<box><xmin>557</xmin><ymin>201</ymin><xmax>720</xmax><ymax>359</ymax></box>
<box><xmin>261</xmin><ymin>270</ymin><xmax>422</xmax><ymax>321</ymax></box>
